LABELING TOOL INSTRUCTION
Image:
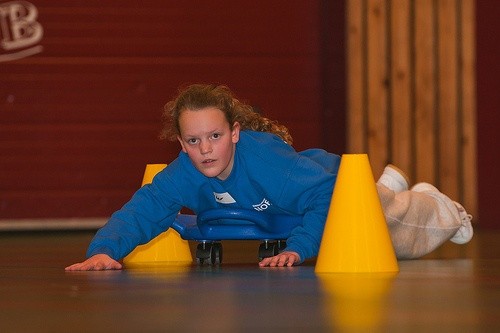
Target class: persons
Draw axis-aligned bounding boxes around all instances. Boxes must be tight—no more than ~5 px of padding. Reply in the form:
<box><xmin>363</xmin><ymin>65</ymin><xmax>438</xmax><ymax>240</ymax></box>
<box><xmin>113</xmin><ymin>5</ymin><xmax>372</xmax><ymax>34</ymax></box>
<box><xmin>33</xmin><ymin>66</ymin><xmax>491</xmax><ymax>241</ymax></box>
<box><xmin>64</xmin><ymin>84</ymin><xmax>473</xmax><ymax>271</ymax></box>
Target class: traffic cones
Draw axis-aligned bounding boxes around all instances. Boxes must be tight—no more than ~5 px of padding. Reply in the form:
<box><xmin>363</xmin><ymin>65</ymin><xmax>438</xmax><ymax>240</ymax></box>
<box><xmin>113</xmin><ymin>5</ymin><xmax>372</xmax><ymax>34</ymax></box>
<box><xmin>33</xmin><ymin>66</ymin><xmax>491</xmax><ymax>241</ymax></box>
<box><xmin>120</xmin><ymin>163</ymin><xmax>193</xmax><ymax>263</ymax></box>
<box><xmin>314</xmin><ymin>154</ymin><xmax>400</xmax><ymax>273</ymax></box>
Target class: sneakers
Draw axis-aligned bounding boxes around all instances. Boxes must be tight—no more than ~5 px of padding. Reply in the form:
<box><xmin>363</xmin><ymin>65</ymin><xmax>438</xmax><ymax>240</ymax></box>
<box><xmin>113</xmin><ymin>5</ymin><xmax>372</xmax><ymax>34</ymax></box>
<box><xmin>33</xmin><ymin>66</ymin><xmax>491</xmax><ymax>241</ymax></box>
<box><xmin>410</xmin><ymin>182</ymin><xmax>474</xmax><ymax>244</ymax></box>
<box><xmin>379</xmin><ymin>164</ymin><xmax>410</xmax><ymax>194</ymax></box>
